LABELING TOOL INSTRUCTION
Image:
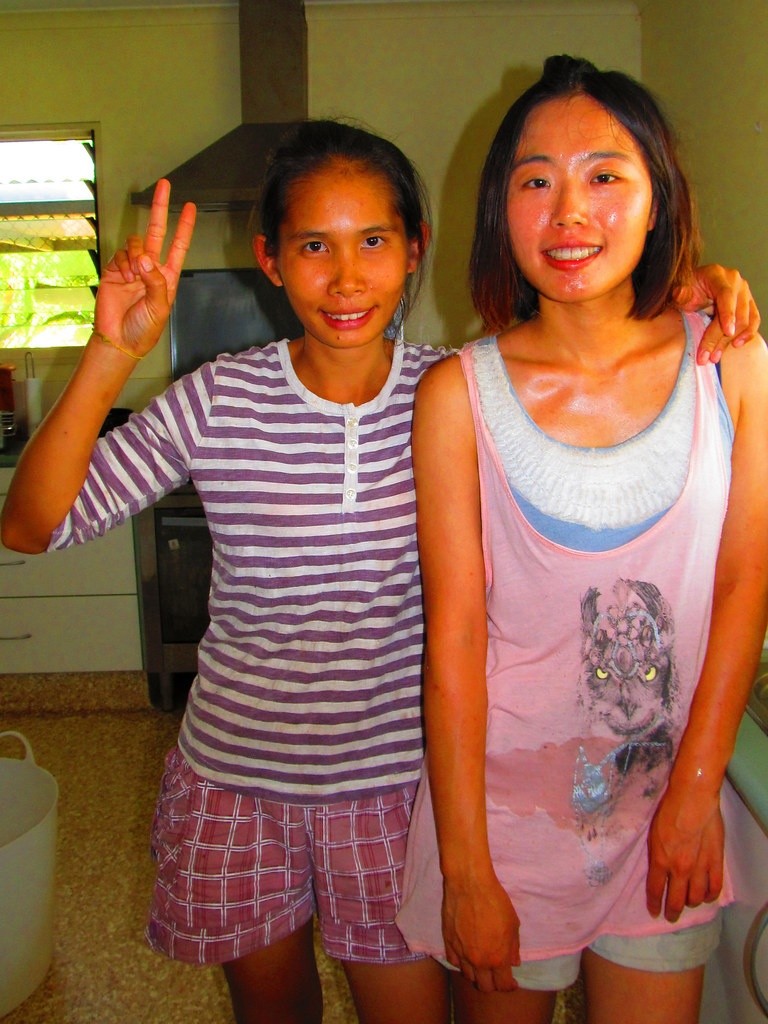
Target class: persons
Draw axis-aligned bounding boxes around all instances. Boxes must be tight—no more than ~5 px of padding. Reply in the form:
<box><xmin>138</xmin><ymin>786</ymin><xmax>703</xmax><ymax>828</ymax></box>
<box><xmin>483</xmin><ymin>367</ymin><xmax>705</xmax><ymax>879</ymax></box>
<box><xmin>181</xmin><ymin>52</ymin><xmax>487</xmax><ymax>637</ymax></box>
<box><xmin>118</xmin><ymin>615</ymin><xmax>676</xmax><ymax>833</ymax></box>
<box><xmin>0</xmin><ymin>114</ymin><xmax>760</xmax><ymax>1023</ymax></box>
<box><xmin>391</xmin><ymin>51</ymin><xmax>768</xmax><ymax>1024</ymax></box>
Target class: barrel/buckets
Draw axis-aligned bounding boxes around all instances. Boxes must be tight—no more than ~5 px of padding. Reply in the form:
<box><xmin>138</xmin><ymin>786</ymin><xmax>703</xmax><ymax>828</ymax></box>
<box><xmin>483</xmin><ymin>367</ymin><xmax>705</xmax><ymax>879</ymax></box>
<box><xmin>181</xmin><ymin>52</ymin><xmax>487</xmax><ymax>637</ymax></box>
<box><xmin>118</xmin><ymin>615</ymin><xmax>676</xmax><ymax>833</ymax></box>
<box><xmin>0</xmin><ymin>731</ymin><xmax>58</xmax><ymax>1020</ymax></box>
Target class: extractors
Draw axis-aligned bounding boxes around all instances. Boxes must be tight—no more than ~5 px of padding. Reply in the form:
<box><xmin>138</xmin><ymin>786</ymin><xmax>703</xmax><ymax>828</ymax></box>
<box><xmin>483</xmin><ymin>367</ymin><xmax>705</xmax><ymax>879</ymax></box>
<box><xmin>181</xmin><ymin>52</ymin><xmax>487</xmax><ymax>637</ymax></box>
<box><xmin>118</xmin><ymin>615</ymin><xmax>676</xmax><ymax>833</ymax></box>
<box><xmin>128</xmin><ymin>123</ymin><xmax>307</xmax><ymax>208</ymax></box>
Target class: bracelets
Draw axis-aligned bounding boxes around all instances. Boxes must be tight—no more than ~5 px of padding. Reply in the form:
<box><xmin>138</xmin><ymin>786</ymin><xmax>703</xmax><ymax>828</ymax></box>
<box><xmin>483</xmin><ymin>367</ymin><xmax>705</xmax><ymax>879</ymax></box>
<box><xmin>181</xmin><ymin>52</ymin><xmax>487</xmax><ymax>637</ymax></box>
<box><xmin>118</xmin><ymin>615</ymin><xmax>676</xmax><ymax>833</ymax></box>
<box><xmin>92</xmin><ymin>330</ymin><xmax>144</xmax><ymax>361</ymax></box>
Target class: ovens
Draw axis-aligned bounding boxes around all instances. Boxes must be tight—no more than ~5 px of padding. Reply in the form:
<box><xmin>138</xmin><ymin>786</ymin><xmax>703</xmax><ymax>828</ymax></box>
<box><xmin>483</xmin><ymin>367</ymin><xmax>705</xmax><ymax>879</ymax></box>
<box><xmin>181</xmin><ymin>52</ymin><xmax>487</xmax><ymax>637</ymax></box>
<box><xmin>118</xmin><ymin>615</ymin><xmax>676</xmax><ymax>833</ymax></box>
<box><xmin>132</xmin><ymin>479</ymin><xmax>215</xmax><ymax>714</ymax></box>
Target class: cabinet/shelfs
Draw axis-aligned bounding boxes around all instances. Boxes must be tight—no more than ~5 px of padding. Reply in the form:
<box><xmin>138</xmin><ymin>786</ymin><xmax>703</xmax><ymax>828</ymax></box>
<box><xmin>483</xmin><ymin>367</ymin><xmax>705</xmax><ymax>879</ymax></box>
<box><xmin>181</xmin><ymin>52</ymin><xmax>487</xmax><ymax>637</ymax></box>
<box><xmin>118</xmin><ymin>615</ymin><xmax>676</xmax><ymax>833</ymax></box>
<box><xmin>0</xmin><ymin>468</ymin><xmax>144</xmax><ymax>674</ymax></box>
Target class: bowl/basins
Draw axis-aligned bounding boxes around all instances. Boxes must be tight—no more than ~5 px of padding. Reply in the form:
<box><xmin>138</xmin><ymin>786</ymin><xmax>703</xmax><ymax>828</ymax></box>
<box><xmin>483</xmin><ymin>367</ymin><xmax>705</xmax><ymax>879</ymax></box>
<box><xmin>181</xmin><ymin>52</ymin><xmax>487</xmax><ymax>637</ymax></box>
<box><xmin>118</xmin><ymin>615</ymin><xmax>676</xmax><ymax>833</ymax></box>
<box><xmin>97</xmin><ymin>408</ymin><xmax>134</xmax><ymax>438</ymax></box>
